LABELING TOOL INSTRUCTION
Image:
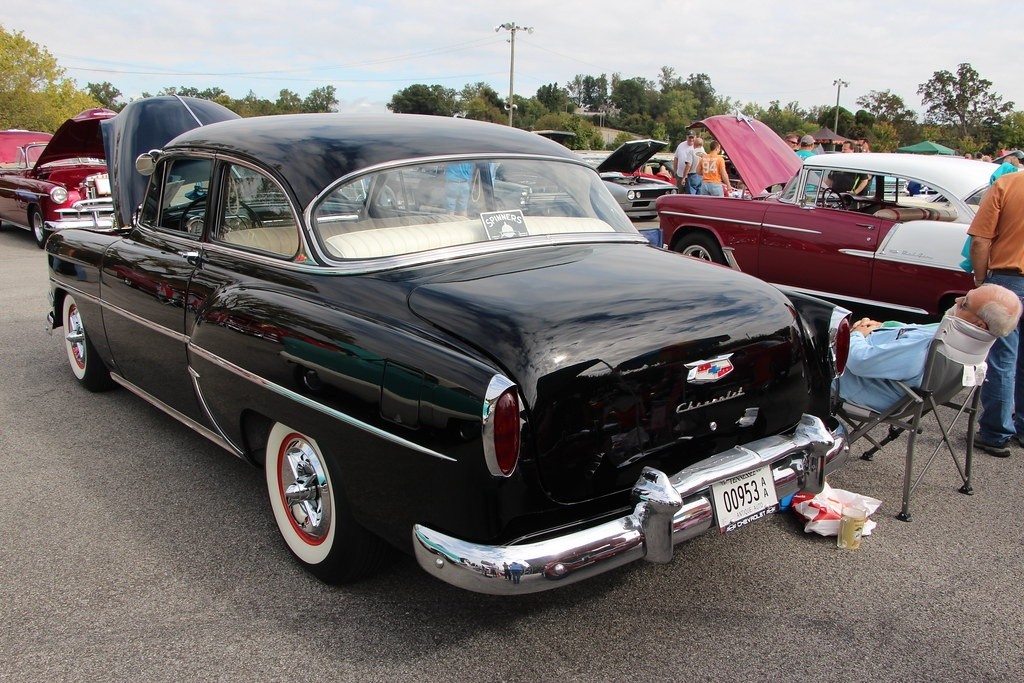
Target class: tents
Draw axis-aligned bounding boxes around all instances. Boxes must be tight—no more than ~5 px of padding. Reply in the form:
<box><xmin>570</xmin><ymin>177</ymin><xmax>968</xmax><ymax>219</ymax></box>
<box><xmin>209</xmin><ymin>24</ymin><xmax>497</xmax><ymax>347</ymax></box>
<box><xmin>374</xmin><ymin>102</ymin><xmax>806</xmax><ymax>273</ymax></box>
<box><xmin>812</xmin><ymin>127</ymin><xmax>857</xmax><ymax>152</ymax></box>
<box><xmin>897</xmin><ymin>141</ymin><xmax>955</xmax><ymax>156</ymax></box>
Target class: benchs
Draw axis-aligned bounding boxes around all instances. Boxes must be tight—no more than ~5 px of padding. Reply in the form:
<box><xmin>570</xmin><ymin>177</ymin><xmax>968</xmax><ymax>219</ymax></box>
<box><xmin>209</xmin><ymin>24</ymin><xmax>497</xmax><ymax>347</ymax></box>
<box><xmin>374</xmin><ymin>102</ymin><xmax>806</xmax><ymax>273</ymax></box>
<box><xmin>872</xmin><ymin>205</ymin><xmax>958</xmax><ymax>222</ymax></box>
<box><xmin>219</xmin><ymin>210</ymin><xmax>617</xmax><ymax>259</ymax></box>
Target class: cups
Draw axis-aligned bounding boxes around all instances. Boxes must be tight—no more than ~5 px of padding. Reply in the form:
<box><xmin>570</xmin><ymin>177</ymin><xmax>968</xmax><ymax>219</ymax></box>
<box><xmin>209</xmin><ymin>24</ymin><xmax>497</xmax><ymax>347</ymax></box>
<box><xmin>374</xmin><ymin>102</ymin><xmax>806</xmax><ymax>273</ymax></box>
<box><xmin>837</xmin><ymin>502</ymin><xmax>867</xmax><ymax>549</ymax></box>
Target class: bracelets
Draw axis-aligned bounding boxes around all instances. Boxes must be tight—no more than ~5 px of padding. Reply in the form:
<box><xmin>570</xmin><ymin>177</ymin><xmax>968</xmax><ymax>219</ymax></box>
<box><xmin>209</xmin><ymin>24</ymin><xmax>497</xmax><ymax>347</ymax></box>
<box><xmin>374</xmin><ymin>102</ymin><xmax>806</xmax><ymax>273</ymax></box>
<box><xmin>974</xmin><ymin>276</ymin><xmax>982</xmax><ymax>283</ymax></box>
<box><xmin>854</xmin><ymin>191</ymin><xmax>857</xmax><ymax>195</ymax></box>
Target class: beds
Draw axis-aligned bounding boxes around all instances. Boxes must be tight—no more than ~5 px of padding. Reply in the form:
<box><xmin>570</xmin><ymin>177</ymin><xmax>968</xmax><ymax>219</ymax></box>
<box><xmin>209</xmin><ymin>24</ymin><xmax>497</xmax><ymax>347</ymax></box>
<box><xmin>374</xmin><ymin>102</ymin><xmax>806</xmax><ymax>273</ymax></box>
<box><xmin>831</xmin><ymin>316</ymin><xmax>997</xmax><ymax>523</ymax></box>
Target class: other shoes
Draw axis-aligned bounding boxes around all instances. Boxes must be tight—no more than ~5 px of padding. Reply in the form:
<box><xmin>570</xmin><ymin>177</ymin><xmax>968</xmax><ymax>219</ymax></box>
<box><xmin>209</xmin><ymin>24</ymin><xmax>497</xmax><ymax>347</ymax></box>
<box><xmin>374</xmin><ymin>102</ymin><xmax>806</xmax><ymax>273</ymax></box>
<box><xmin>1013</xmin><ymin>433</ymin><xmax>1024</xmax><ymax>447</ymax></box>
<box><xmin>966</xmin><ymin>430</ymin><xmax>1011</xmax><ymax>456</ymax></box>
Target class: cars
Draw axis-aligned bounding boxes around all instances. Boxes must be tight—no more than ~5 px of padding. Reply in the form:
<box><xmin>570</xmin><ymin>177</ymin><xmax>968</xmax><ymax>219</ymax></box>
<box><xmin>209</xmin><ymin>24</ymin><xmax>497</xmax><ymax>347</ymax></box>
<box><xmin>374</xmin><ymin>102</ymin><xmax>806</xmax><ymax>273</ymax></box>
<box><xmin>414</xmin><ymin>529</ymin><xmax>461</xmax><ymax>565</ymax></box>
<box><xmin>46</xmin><ymin>94</ymin><xmax>855</xmax><ymax>598</ymax></box>
<box><xmin>0</xmin><ymin>106</ymin><xmax>118</xmax><ymax>248</ymax></box>
<box><xmin>528</xmin><ymin>130</ymin><xmax>948</xmax><ymax>221</ymax></box>
<box><xmin>542</xmin><ymin>529</ymin><xmax>643</xmax><ymax>581</ymax></box>
<box><xmin>155</xmin><ymin>170</ymin><xmax>587</xmax><ymax>239</ymax></box>
<box><xmin>657</xmin><ymin>110</ymin><xmax>1002</xmax><ymax>321</ymax></box>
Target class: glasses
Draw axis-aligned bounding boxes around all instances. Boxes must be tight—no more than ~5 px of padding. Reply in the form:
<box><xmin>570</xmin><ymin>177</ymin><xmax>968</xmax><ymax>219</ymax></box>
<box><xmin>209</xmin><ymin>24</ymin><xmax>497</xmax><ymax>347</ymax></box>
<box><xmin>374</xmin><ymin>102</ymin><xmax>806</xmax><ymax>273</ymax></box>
<box><xmin>787</xmin><ymin>139</ymin><xmax>799</xmax><ymax>145</ymax></box>
<box><xmin>960</xmin><ymin>297</ymin><xmax>986</xmax><ymax>323</ymax></box>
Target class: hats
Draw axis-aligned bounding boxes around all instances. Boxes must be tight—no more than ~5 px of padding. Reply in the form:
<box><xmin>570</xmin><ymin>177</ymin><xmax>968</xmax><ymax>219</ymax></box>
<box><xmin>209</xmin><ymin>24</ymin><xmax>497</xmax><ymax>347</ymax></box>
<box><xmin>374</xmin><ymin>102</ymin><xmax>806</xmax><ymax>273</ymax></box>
<box><xmin>801</xmin><ymin>135</ymin><xmax>815</xmax><ymax>145</ymax></box>
<box><xmin>687</xmin><ymin>130</ymin><xmax>696</xmax><ymax>137</ymax></box>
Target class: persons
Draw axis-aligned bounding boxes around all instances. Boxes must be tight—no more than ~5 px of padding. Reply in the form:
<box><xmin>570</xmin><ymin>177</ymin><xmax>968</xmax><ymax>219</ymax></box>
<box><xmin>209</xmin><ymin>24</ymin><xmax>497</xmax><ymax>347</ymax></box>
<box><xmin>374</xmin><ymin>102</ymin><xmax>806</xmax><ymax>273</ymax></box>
<box><xmin>781</xmin><ymin>134</ymin><xmax>823</xmax><ymax>192</ymax></box>
<box><xmin>444</xmin><ymin>162</ymin><xmax>500</xmax><ymax>216</ymax></box>
<box><xmin>966</xmin><ymin>169</ymin><xmax>1024</xmax><ymax>457</ymax></box>
<box><xmin>966</xmin><ymin>148</ymin><xmax>1023</xmax><ymax>168</ymax></box>
<box><xmin>673</xmin><ymin>130</ymin><xmax>731</xmax><ymax>197</ymax></box>
<box><xmin>825</xmin><ymin>138</ymin><xmax>875</xmax><ymax>196</ymax></box>
<box><xmin>832</xmin><ymin>283</ymin><xmax>1023</xmax><ymax>414</ymax></box>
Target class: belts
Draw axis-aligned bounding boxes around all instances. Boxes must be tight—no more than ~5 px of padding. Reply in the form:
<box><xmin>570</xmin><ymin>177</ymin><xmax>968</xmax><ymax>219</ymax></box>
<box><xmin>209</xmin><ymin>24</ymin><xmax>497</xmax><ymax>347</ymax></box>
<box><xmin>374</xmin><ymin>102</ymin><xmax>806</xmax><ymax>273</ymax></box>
<box><xmin>993</xmin><ymin>269</ymin><xmax>1018</xmax><ymax>277</ymax></box>
<box><xmin>449</xmin><ymin>180</ymin><xmax>467</xmax><ymax>184</ymax></box>
